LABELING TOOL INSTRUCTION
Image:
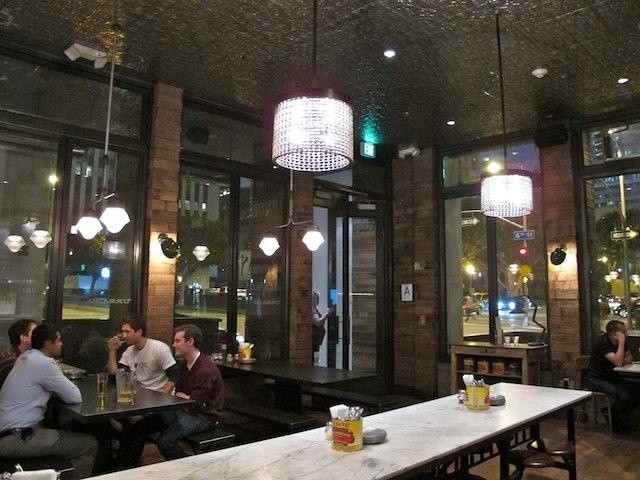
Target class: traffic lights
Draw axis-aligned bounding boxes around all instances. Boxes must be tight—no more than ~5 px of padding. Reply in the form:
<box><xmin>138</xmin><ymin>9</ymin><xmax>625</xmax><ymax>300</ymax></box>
<box><xmin>518</xmin><ymin>248</ymin><xmax>529</xmax><ymax>262</ymax></box>
<box><xmin>521</xmin><ymin>276</ymin><xmax>529</xmax><ymax>284</ymax></box>
<box><xmin>593</xmin><ymin>129</ymin><xmax>611</xmax><ymax>159</ymax></box>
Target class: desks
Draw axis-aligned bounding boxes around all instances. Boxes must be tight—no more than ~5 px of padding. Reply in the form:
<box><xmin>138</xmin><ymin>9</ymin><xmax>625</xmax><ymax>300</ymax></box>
<box><xmin>58</xmin><ymin>375</ymin><xmax>598</xmax><ymax>479</ymax></box>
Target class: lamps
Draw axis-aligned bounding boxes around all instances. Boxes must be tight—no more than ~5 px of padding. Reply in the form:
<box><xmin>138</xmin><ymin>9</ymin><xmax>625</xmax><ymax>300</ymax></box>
<box><xmin>476</xmin><ymin>0</ymin><xmax>537</xmax><ymax>224</ymax></box>
<box><xmin>271</xmin><ymin>0</ymin><xmax>358</xmax><ymax>180</ymax></box>
<box><xmin>193</xmin><ymin>244</ymin><xmax>214</xmax><ymax>262</ymax></box>
<box><xmin>76</xmin><ymin>0</ymin><xmax>130</xmax><ymax>242</ymax></box>
<box><xmin>3</xmin><ymin>120</ymin><xmax>54</xmax><ymax>256</ymax></box>
<box><xmin>256</xmin><ymin>15</ymin><xmax>325</xmax><ymax>257</ymax></box>
<box><xmin>549</xmin><ymin>244</ymin><xmax>569</xmax><ymax>267</ymax></box>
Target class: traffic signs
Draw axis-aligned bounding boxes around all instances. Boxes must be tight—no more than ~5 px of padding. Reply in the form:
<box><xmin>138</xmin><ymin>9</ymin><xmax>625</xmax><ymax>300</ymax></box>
<box><xmin>512</xmin><ymin>230</ymin><xmax>536</xmax><ymax>239</ymax></box>
<box><xmin>610</xmin><ymin>230</ymin><xmax>640</xmax><ymax>241</ymax></box>
<box><xmin>461</xmin><ymin>217</ymin><xmax>480</xmax><ymax>227</ymax></box>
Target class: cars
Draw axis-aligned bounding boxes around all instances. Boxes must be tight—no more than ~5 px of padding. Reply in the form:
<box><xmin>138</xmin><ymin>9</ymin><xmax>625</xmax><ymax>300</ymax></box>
<box><xmin>205</xmin><ymin>286</ymin><xmax>247</xmax><ymax>301</ymax></box>
<box><xmin>462</xmin><ymin>292</ymin><xmax>529</xmax><ymax>315</ymax></box>
<box><xmin>598</xmin><ymin>295</ymin><xmax>639</xmax><ymax>318</ymax></box>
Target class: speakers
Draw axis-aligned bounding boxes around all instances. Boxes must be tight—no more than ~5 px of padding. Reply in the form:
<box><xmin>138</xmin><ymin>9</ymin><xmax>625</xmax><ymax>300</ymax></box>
<box><xmin>187</xmin><ymin>127</ymin><xmax>209</xmax><ymax>145</ymax></box>
<box><xmin>534</xmin><ymin>123</ymin><xmax>569</xmax><ymax>149</ymax></box>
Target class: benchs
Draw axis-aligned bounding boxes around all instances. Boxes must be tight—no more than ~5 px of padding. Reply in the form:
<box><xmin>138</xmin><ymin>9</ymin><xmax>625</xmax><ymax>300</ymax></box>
<box><xmin>217</xmin><ymin>393</ymin><xmax>315</xmax><ymax>434</ymax></box>
<box><xmin>188</xmin><ymin>426</ymin><xmax>237</xmax><ymax>453</ymax></box>
<box><xmin>307</xmin><ymin>382</ymin><xmax>398</xmax><ymax>411</ymax></box>
<box><xmin>4</xmin><ymin>449</ymin><xmax>78</xmax><ymax>479</ymax></box>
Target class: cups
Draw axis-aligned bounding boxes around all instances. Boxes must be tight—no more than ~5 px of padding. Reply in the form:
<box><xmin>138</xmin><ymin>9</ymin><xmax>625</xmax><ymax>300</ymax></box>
<box><xmin>504</xmin><ymin>335</ymin><xmax>512</xmax><ymax>346</ymax></box>
<box><xmin>94</xmin><ymin>371</ymin><xmax>110</xmax><ymax>401</ymax></box>
<box><xmin>108</xmin><ymin>334</ymin><xmax>126</xmax><ymax>348</ymax></box>
<box><xmin>513</xmin><ymin>335</ymin><xmax>521</xmax><ymax>346</ymax></box>
<box><xmin>113</xmin><ymin>370</ymin><xmax>138</xmax><ymax>404</ymax></box>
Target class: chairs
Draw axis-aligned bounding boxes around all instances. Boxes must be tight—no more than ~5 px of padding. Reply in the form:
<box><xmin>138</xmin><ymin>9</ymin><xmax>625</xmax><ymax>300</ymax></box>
<box><xmin>577</xmin><ymin>353</ymin><xmax>613</xmax><ymax>434</ymax></box>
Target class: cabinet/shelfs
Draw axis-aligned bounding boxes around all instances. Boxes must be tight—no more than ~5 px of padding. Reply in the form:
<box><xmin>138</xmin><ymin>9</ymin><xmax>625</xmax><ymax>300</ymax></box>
<box><xmin>449</xmin><ymin>338</ymin><xmax>549</xmax><ymax>394</ymax></box>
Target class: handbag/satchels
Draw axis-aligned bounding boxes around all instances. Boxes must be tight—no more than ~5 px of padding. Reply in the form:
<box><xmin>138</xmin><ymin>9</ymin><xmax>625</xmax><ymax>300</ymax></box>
<box><xmin>311</xmin><ymin>324</ymin><xmax>326</xmax><ymax>346</ymax></box>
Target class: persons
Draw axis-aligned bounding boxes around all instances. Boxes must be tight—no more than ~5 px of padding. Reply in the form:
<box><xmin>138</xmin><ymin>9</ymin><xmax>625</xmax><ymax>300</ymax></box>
<box><xmin>114</xmin><ymin>323</ymin><xmax>225</xmax><ymax>469</ymax></box>
<box><xmin>312</xmin><ymin>290</ymin><xmax>335</xmax><ymax>365</ymax></box>
<box><xmin>462</xmin><ymin>292</ymin><xmax>475</xmax><ymax>322</ymax></box>
<box><xmin>585</xmin><ymin>319</ymin><xmax>636</xmax><ymax>433</ymax></box>
<box><xmin>1</xmin><ymin>323</ymin><xmax>102</xmax><ymax>480</ymax></box>
<box><xmin>105</xmin><ymin>315</ymin><xmax>180</xmax><ymax>472</ymax></box>
<box><xmin>0</xmin><ymin>317</ymin><xmax>40</xmax><ymax>391</ymax></box>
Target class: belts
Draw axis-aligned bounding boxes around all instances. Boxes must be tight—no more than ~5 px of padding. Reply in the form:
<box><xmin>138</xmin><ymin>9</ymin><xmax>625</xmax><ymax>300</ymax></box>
<box><xmin>0</xmin><ymin>427</ymin><xmax>24</xmax><ymax>439</ymax></box>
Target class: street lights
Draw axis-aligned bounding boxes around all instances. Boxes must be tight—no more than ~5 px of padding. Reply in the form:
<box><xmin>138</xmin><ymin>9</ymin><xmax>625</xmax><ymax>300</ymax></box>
<box><xmin>465</xmin><ymin>265</ymin><xmax>476</xmax><ymax>292</ymax></box>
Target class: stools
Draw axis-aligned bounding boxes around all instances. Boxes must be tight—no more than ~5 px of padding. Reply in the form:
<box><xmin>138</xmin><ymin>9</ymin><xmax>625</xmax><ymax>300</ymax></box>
<box><xmin>503</xmin><ymin>447</ymin><xmax>559</xmax><ymax>473</ymax></box>
<box><xmin>526</xmin><ymin>434</ymin><xmax>578</xmax><ymax>459</ymax></box>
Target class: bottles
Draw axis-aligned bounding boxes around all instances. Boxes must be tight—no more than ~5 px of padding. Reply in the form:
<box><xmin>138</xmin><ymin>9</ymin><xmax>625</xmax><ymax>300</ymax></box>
<box><xmin>210</xmin><ymin>352</ymin><xmax>241</xmax><ymax>364</ymax></box>
